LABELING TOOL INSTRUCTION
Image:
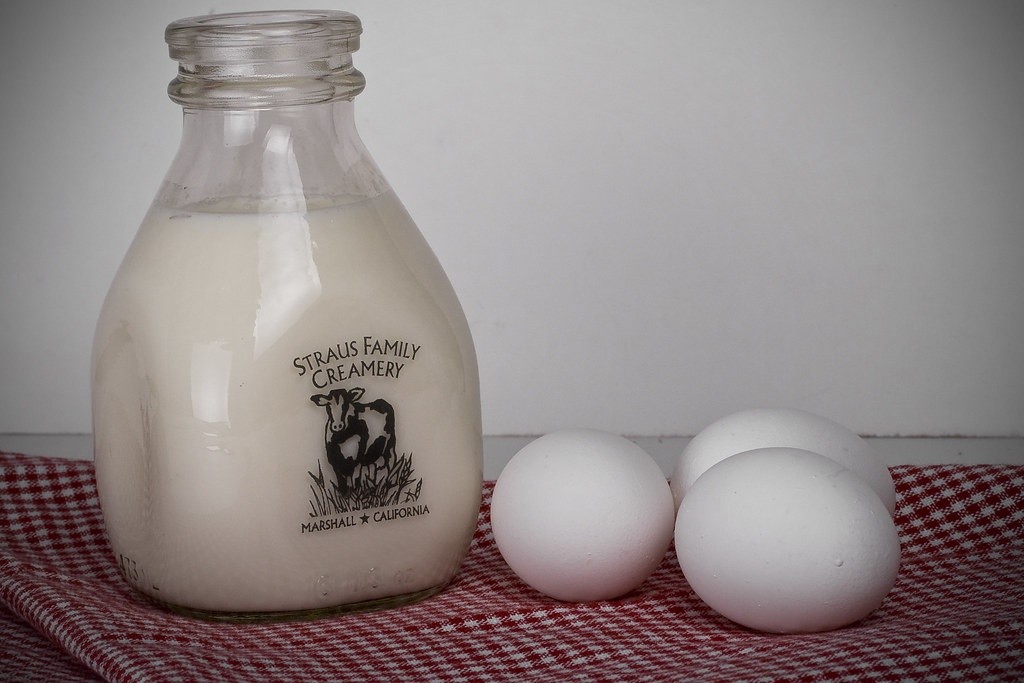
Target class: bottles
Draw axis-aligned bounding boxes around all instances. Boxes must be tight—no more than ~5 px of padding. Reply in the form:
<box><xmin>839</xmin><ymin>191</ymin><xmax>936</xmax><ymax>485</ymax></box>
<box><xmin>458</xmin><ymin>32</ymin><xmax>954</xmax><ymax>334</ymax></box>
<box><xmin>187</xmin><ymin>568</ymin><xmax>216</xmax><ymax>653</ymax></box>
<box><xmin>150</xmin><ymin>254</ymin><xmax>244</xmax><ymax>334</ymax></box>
<box><xmin>94</xmin><ymin>10</ymin><xmax>483</xmax><ymax>622</ymax></box>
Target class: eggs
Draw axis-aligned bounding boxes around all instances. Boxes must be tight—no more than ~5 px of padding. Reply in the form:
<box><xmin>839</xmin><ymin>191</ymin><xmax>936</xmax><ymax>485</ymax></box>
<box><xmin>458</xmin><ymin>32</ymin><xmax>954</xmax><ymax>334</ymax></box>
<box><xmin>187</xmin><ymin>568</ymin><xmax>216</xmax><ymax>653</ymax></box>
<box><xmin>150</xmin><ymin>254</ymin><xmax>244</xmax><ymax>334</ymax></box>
<box><xmin>673</xmin><ymin>446</ymin><xmax>900</xmax><ymax>634</ymax></box>
<box><xmin>491</xmin><ymin>428</ymin><xmax>676</xmax><ymax>603</ymax></box>
<box><xmin>669</xmin><ymin>408</ymin><xmax>896</xmax><ymax>518</ymax></box>
